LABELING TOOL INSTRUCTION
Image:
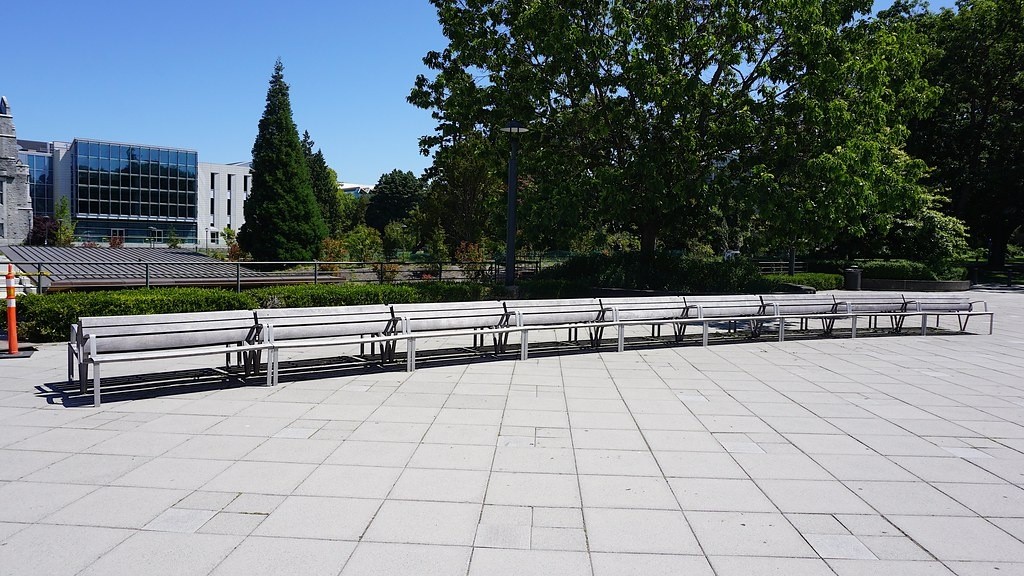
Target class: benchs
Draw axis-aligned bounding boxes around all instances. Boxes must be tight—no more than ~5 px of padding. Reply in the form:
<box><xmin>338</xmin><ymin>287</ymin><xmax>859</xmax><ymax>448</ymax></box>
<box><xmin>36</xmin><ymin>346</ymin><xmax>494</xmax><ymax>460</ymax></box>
<box><xmin>67</xmin><ymin>290</ymin><xmax>992</xmax><ymax>408</ymax></box>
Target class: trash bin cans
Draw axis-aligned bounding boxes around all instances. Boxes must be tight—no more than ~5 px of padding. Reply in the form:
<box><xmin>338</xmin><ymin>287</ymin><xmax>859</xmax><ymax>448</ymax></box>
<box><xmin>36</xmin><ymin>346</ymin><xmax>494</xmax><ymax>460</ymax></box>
<box><xmin>843</xmin><ymin>268</ymin><xmax>863</xmax><ymax>290</ymax></box>
